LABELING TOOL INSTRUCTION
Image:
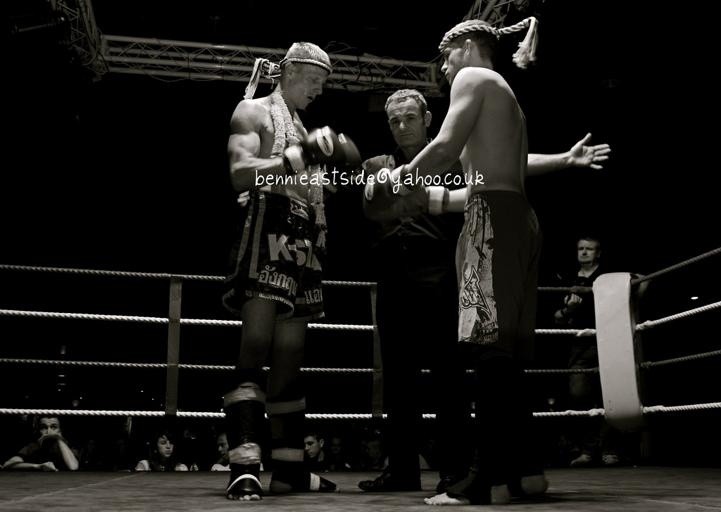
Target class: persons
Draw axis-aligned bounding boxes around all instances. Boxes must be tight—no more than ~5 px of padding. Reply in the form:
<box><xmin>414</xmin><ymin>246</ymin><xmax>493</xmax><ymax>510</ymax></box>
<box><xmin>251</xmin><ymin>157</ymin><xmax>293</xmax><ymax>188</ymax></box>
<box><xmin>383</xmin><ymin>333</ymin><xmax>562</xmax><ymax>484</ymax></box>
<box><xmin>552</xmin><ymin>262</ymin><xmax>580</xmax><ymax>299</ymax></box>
<box><xmin>357</xmin><ymin>87</ymin><xmax>613</xmax><ymax>496</ymax></box>
<box><xmin>545</xmin><ymin>235</ymin><xmax>654</xmax><ymax>467</ymax></box>
<box><xmin>1</xmin><ymin>412</ymin><xmax>387</xmax><ymax>475</ymax></box>
<box><xmin>360</xmin><ymin>16</ymin><xmax>547</xmax><ymax>507</ymax></box>
<box><xmin>222</xmin><ymin>39</ymin><xmax>363</xmax><ymax>503</ymax></box>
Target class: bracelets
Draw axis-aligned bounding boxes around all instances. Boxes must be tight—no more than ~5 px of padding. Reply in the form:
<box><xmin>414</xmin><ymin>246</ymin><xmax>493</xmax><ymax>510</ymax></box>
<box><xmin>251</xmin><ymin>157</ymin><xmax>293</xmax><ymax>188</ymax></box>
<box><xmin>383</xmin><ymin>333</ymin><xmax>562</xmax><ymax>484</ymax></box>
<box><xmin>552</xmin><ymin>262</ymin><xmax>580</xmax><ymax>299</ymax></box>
<box><xmin>560</xmin><ymin>309</ymin><xmax>566</xmax><ymax>317</ymax></box>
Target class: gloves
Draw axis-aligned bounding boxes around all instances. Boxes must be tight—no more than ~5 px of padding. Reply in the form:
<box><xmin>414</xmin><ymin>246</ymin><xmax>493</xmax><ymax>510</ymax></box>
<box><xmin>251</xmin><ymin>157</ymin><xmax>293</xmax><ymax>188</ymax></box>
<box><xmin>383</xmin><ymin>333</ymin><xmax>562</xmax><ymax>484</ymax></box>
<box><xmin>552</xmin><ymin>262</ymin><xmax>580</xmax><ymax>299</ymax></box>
<box><xmin>283</xmin><ymin>125</ymin><xmax>449</xmax><ymax>220</ymax></box>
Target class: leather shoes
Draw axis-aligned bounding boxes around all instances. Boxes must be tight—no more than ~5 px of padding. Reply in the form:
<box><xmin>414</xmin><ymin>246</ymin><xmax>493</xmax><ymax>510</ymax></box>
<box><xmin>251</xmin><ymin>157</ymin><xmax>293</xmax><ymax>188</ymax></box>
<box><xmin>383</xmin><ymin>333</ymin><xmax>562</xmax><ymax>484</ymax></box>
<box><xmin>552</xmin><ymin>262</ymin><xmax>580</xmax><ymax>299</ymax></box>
<box><xmin>358</xmin><ymin>465</ymin><xmax>421</xmax><ymax>492</ymax></box>
